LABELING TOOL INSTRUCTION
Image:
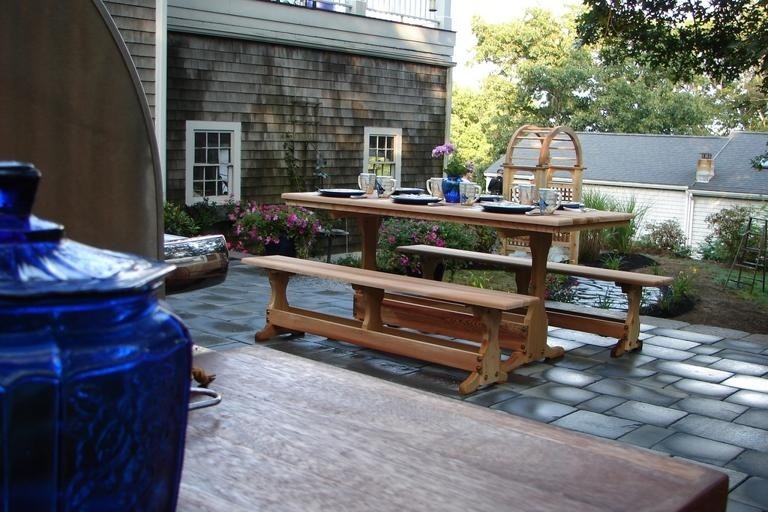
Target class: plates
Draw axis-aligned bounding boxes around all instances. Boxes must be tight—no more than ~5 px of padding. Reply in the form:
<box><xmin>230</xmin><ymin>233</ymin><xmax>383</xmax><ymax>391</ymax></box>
<box><xmin>558</xmin><ymin>201</ymin><xmax>582</xmax><ymax>208</ymax></box>
<box><xmin>474</xmin><ymin>195</ymin><xmax>504</xmax><ymax>201</ymax></box>
<box><xmin>319</xmin><ymin>188</ymin><xmax>367</xmax><ymax>198</ymax></box>
<box><xmin>481</xmin><ymin>200</ymin><xmax>535</xmax><ymax>214</ymax></box>
<box><xmin>389</xmin><ymin>186</ymin><xmax>443</xmax><ymax>204</ymax></box>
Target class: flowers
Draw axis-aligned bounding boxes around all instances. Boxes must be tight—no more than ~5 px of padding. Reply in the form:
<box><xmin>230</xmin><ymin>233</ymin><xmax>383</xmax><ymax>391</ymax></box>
<box><xmin>215</xmin><ymin>197</ymin><xmax>327</xmax><ymax>259</ymax></box>
<box><xmin>370</xmin><ymin>215</ymin><xmax>499</xmax><ymax>279</ymax></box>
<box><xmin>429</xmin><ymin>141</ymin><xmax>476</xmax><ymax>179</ymax></box>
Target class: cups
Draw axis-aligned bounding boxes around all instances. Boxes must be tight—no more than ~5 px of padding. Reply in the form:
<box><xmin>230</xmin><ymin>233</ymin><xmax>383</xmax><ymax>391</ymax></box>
<box><xmin>375</xmin><ymin>176</ymin><xmax>396</xmax><ymax>198</ymax></box>
<box><xmin>538</xmin><ymin>188</ymin><xmax>562</xmax><ymax>214</ymax></box>
<box><xmin>442</xmin><ymin>179</ymin><xmax>461</xmax><ymax>203</ymax></box>
<box><xmin>512</xmin><ymin>185</ymin><xmax>535</xmax><ymax>205</ymax></box>
<box><xmin>426</xmin><ymin>178</ymin><xmax>444</xmax><ymax>200</ymax></box>
<box><xmin>357</xmin><ymin>173</ymin><xmax>376</xmax><ymax>194</ymax></box>
<box><xmin>459</xmin><ymin>182</ymin><xmax>482</xmax><ymax>206</ymax></box>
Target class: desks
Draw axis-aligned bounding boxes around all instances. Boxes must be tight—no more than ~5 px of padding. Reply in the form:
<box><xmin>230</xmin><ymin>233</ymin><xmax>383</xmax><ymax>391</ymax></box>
<box><xmin>275</xmin><ymin>183</ymin><xmax>642</xmax><ymax>381</ymax></box>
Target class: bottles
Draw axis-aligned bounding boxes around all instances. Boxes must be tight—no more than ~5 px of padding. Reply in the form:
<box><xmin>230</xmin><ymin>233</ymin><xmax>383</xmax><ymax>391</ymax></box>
<box><xmin>0</xmin><ymin>161</ymin><xmax>193</xmax><ymax>512</ymax></box>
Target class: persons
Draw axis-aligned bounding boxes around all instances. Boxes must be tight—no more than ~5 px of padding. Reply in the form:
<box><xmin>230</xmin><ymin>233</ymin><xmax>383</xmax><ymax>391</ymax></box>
<box><xmin>487</xmin><ymin>168</ymin><xmax>503</xmax><ymax>196</ymax></box>
<box><xmin>461</xmin><ymin>168</ymin><xmax>473</xmax><ymax>183</ymax></box>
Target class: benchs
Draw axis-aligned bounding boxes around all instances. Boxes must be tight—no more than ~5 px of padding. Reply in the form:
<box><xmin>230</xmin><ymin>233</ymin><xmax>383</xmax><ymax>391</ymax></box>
<box><xmin>234</xmin><ymin>248</ymin><xmax>568</xmax><ymax>401</ymax></box>
<box><xmin>394</xmin><ymin>235</ymin><xmax>674</xmax><ymax>365</ymax></box>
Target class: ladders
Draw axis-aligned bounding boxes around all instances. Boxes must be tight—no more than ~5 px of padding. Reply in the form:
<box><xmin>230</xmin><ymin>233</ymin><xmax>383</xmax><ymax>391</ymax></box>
<box><xmin>724</xmin><ymin>216</ymin><xmax>768</xmax><ymax>294</ymax></box>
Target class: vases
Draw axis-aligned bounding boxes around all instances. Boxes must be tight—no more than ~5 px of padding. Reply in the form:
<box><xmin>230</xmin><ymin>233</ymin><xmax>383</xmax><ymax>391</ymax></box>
<box><xmin>404</xmin><ymin>259</ymin><xmax>445</xmax><ymax>284</ymax></box>
<box><xmin>257</xmin><ymin>233</ymin><xmax>318</xmax><ymax>268</ymax></box>
<box><xmin>439</xmin><ymin>173</ymin><xmax>468</xmax><ymax>204</ymax></box>
